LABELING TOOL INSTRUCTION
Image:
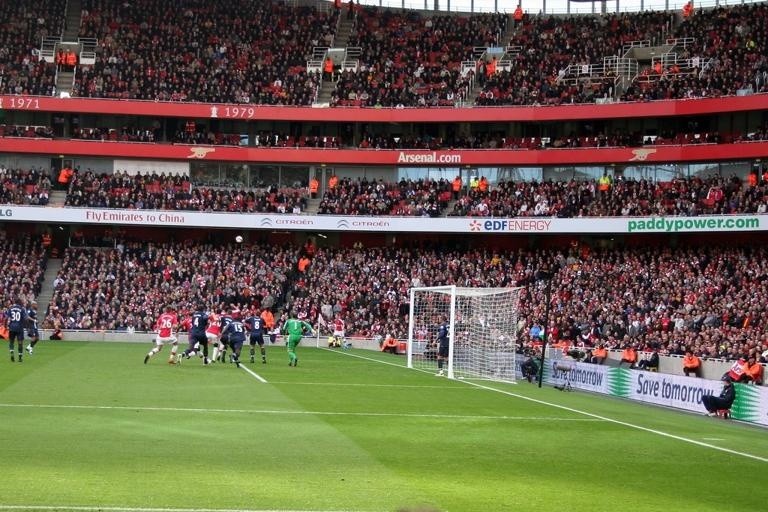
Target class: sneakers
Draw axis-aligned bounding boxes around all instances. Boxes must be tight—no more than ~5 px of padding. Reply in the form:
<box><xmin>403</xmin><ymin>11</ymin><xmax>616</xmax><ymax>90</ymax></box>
<box><xmin>435</xmin><ymin>369</ymin><xmax>445</xmax><ymax>377</ymax></box>
<box><xmin>705</xmin><ymin>411</ymin><xmax>720</xmax><ymax>418</ymax></box>
<box><xmin>142</xmin><ymin>351</ymin><xmax>299</xmax><ymax>366</ymax></box>
<box><xmin>8</xmin><ymin>346</ymin><xmax>34</xmax><ymax>363</ymax></box>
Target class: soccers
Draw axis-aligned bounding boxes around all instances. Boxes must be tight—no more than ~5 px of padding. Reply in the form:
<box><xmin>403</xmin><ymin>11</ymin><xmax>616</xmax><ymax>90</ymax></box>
<box><xmin>236</xmin><ymin>236</ymin><xmax>243</xmax><ymax>243</ymax></box>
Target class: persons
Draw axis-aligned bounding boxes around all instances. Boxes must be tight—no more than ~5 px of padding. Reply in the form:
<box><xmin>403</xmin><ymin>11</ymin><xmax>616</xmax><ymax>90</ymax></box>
<box><xmin>0</xmin><ymin>0</ymin><xmax>768</xmax><ymax>384</ymax></box>
<box><xmin>701</xmin><ymin>376</ymin><xmax>736</xmax><ymax>417</ymax></box>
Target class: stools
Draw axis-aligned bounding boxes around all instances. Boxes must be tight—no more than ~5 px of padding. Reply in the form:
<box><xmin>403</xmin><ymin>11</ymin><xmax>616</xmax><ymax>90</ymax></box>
<box><xmin>716</xmin><ymin>408</ymin><xmax>731</xmax><ymax>420</ymax></box>
<box><xmin>646</xmin><ymin>366</ymin><xmax>658</xmax><ymax>372</ymax></box>
